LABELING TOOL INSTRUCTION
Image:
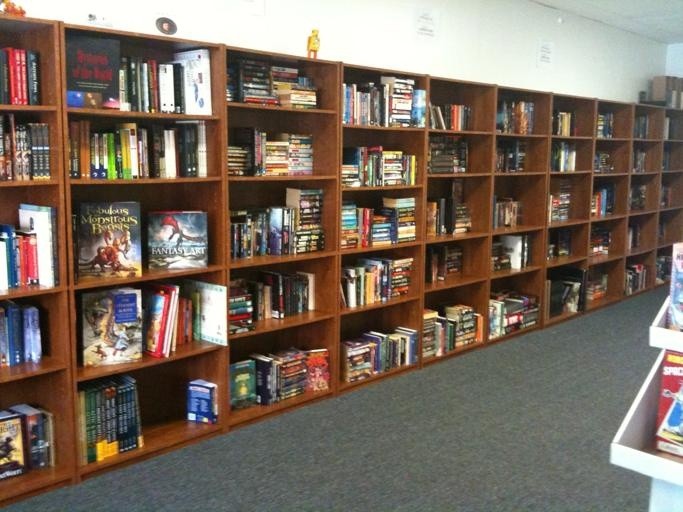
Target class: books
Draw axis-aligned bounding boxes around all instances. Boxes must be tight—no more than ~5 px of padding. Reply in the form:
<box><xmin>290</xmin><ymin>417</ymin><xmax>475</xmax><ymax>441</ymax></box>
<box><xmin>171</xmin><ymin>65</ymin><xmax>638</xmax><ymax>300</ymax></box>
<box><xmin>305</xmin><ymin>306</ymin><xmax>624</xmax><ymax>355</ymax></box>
<box><xmin>588</xmin><ymin>112</ymin><xmax>613</xmax><ymax>300</ymax></box>
<box><xmin>624</xmin><ymin>113</ymin><xmax>673</xmax><ymax>296</ymax></box>
<box><xmin>546</xmin><ymin>110</ymin><xmax>587</xmax><ymax>315</ymax></box>
<box><xmin>656</xmin><ymin>351</ymin><xmax>683</xmax><ymax>457</ymax></box>
<box><xmin>64</xmin><ymin>43</ymin><xmax>228</xmax><ymax>464</ymax></box>
<box><xmin>490</xmin><ymin>99</ymin><xmax>539</xmax><ymax>340</ymax></box>
<box><xmin>422</xmin><ymin>101</ymin><xmax>484</xmax><ymax>359</ymax></box>
<box><xmin>341</xmin><ymin>75</ymin><xmax>426</xmax><ymax>384</ymax></box>
<box><xmin>666</xmin><ymin>243</ymin><xmax>683</xmax><ymax>329</ymax></box>
<box><xmin>224</xmin><ymin>55</ymin><xmax>332</xmax><ymax>411</ymax></box>
<box><xmin>0</xmin><ymin>47</ymin><xmax>54</xmax><ymax>478</ymax></box>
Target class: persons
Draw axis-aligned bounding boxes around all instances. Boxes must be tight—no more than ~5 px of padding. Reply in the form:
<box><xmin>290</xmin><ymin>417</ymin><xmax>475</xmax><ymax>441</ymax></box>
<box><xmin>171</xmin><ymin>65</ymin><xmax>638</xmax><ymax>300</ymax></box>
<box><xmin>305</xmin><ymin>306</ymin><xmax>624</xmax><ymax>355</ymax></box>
<box><xmin>308</xmin><ymin>28</ymin><xmax>321</xmax><ymax>59</ymax></box>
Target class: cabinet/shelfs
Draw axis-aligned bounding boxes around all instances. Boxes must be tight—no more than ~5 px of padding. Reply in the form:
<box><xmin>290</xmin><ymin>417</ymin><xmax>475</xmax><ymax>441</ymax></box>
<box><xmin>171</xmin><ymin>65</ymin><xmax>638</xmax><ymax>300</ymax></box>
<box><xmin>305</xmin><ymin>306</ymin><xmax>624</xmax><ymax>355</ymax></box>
<box><xmin>609</xmin><ymin>296</ymin><xmax>683</xmax><ymax>511</ymax></box>
<box><xmin>0</xmin><ymin>11</ymin><xmax>683</xmax><ymax>507</ymax></box>
